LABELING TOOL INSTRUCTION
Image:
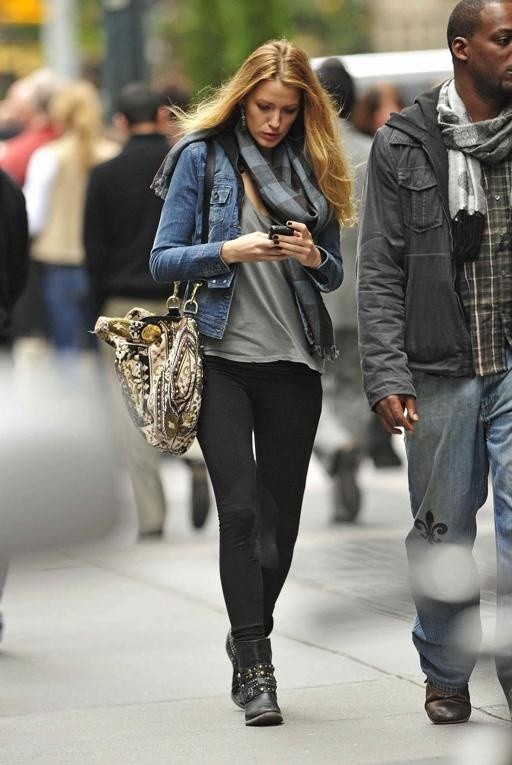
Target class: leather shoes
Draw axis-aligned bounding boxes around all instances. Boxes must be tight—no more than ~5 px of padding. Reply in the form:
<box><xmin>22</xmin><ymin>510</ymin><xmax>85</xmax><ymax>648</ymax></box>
<box><xmin>423</xmin><ymin>677</ymin><xmax>472</xmax><ymax>724</ymax></box>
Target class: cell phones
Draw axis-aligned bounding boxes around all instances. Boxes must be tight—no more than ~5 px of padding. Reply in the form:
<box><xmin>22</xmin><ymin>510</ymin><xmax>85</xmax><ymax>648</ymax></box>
<box><xmin>267</xmin><ymin>224</ymin><xmax>296</xmax><ymax>244</ymax></box>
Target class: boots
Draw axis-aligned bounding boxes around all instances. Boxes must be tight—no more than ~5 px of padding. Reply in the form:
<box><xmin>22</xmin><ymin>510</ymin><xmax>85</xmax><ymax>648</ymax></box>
<box><xmin>223</xmin><ymin>625</ymin><xmax>284</xmax><ymax>726</ymax></box>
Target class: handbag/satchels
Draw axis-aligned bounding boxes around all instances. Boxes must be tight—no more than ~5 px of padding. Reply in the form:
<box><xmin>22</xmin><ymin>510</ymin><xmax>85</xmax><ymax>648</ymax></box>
<box><xmin>87</xmin><ymin>295</ymin><xmax>204</xmax><ymax>458</ymax></box>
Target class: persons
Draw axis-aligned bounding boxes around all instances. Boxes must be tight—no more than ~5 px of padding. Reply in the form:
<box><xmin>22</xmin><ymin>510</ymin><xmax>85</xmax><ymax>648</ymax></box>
<box><xmin>2</xmin><ymin>56</ymin><xmax>212</xmax><ymax>537</ymax></box>
<box><xmin>148</xmin><ymin>0</ymin><xmax>512</xmax><ymax>725</ymax></box>
